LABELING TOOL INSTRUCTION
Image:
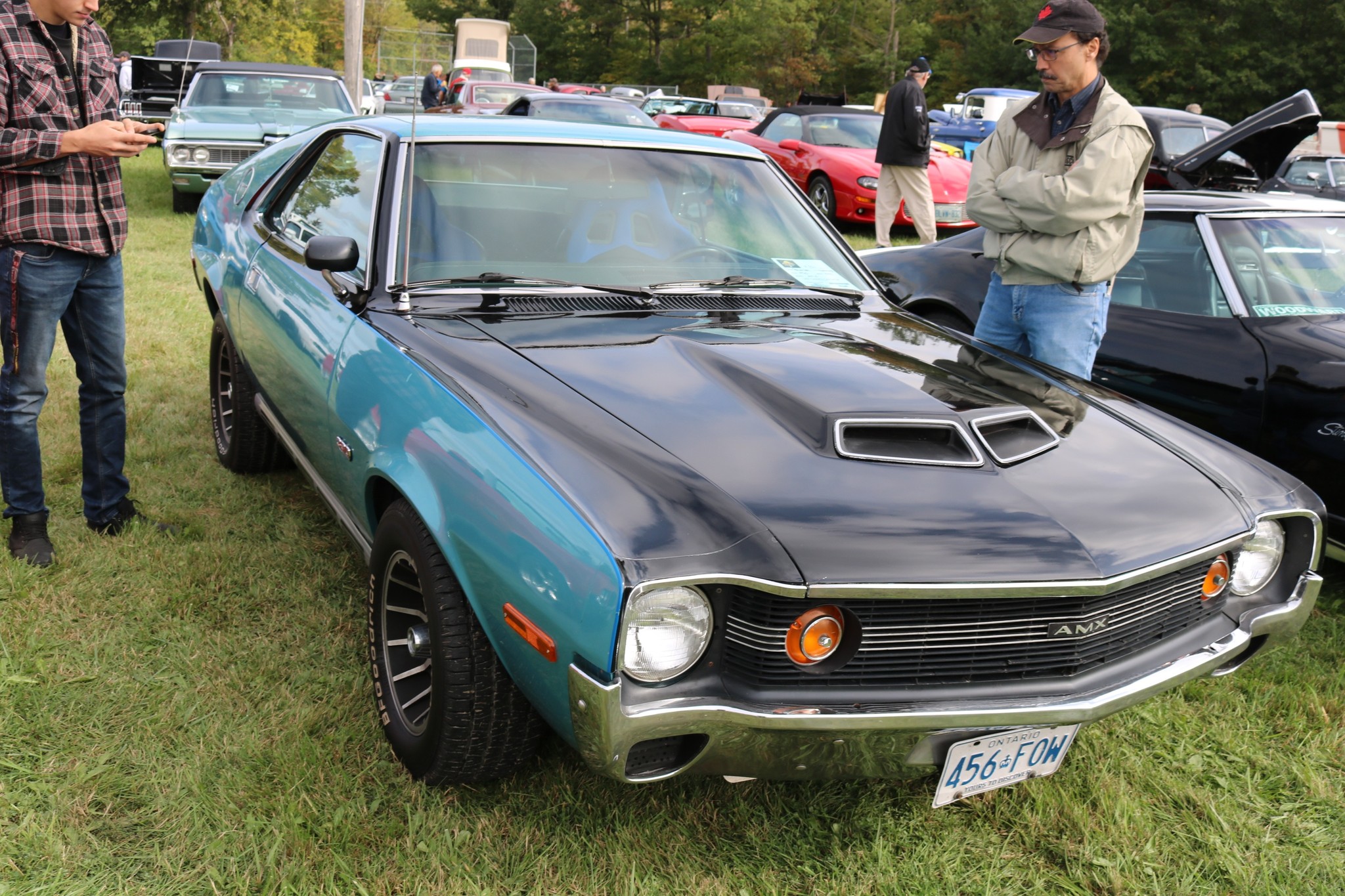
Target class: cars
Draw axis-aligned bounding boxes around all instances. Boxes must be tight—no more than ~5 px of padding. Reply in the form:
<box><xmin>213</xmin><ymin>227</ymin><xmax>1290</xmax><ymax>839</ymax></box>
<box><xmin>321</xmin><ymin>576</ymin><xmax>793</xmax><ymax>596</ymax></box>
<box><xmin>853</xmin><ymin>192</ymin><xmax>1345</xmax><ymax>566</ymax></box>
<box><xmin>161</xmin><ymin>61</ymin><xmax>357</xmax><ymax>214</ymax></box>
<box><xmin>340</xmin><ymin>58</ymin><xmax>1345</xmax><ymax>230</ymax></box>
<box><xmin>189</xmin><ymin>116</ymin><xmax>1326</xmax><ymax>808</ymax></box>
<box><xmin>116</xmin><ymin>41</ymin><xmax>221</xmax><ymax>138</ymax></box>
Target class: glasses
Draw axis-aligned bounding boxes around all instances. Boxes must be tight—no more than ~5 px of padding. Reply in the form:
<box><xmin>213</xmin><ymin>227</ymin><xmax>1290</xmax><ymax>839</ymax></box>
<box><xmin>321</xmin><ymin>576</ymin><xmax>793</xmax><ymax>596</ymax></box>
<box><xmin>1026</xmin><ymin>42</ymin><xmax>1081</xmax><ymax>61</ymax></box>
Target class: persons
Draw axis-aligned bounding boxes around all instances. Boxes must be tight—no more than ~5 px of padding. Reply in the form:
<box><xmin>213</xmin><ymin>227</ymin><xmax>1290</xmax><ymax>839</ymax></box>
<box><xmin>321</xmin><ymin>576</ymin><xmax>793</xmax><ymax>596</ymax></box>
<box><xmin>421</xmin><ymin>64</ymin><xmax>446</xmax><ymax>110</ymax></box>
<box><xmin>965</xmin><ymin>0</ymin><xmax>1155</xmax><ymax>381</ymax></box>
<box><xmin>921</xmin><ymin>342</ymin><xmax>1137</xmax><ymax>438</ymax></box>
<box><xmin>321</xmin><ymin>334</ymin><xmax>486</xmax><ymax>448</ymax></box>
<box><xmin>449</xmin><ymin>68</ymin><xmax>472</xmax><ymax>103</ymax></box>
<box><xmin>874</xmin><ymin>56</ymin><xmax>937</xmax><ymax>248</ymax></box>
<box><xmin>1185</xmin><ymin>103</ymin><xmax>1202</xmax><ymax>114</ymax></box>
<box><xmin>547</xmin><ymin>78</ymin><xmax>559</xmax><ymax>92</ymax></box>
<box><xmin>528</xmin><ymin>77</ymin><xmax>536</xmax><ymax>85</ymax></box>
<box><xmin>0</xmin><ymin>0</ymin><xmax>178</xmax><ymax>567</ymax></box>
<box><xmin>436</xmin><ymin>73</ymin><xmax>447</xmax><ymax>105</ymax></box>
<box><xmin>599</xmin><ymin>85</ymin><xmax>610</xmax><ymax>94</ymax></box>
<box><xmin>373</xmin><ymin>73</ymin><xmax>398</xmax><ymax>82</ymax></box>
<box><xmin>783</xmin><ymin>101</ymin><xmax>792</xmax><ymax>107</ymax></box>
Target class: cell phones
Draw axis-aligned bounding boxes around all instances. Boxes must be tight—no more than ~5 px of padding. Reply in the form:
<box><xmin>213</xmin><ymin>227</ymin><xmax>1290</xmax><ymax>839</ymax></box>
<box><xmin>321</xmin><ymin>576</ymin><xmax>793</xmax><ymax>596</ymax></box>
<box><xmin>139</xmin><ymin>127</ymin><xmax>162</xmax><ymax>136</ymax></box>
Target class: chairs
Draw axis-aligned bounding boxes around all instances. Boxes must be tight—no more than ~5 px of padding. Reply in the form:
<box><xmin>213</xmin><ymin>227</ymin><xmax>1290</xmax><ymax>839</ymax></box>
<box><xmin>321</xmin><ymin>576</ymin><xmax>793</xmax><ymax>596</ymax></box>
<box><xmin>552</xmin><ymin>168</ymin><xmax>700</xmax><ymax>264</ymax></box>
<box><xmin>325</xmin><ymin>170</ymin><xmax>487</xmax><ymax>273</ymax></box>
<box><xmin>1111</xmin><ymin>255</ymin><xmax>1158</xmax><ymax>310</ymax></box>
<box><xmin>1199</xmin><ymin>243</ymin><xmax>1271</xmax><ymax>317</ymax></box>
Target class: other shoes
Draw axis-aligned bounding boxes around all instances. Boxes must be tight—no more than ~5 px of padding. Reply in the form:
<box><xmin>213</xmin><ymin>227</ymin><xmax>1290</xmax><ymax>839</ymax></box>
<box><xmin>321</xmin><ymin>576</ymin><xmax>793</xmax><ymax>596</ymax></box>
<box><xmin>9</xmin><ymin>510</ymin><xmax>58</xmax><ymax>568</ymax></box>
<box><xmin>87</xmin><ymin>495</ymin><xmax>175</xmax><ymax>540</ymax></box>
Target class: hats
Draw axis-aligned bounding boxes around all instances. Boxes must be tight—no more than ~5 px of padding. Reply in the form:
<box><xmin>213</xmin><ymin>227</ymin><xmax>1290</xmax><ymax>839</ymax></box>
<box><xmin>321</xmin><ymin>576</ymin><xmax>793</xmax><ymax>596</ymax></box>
<box><xmin>1012</xmin><ymin>0</ymin><xmax>1106</xmax><ymax>45</ymax></box>
<box><xmin>463</xmin><ymin>68</ymin><xmax>472</xmax><ymax>75</ymax></box>
<box><xmin>904</xmin><ymin>57</ymin><xmax>932</xmax><ymax>74</ymax></box>
<box><xmin>550</xmin><ymin>78</ymin><xmax>557</xmax><ymax>83</ymax></box>
